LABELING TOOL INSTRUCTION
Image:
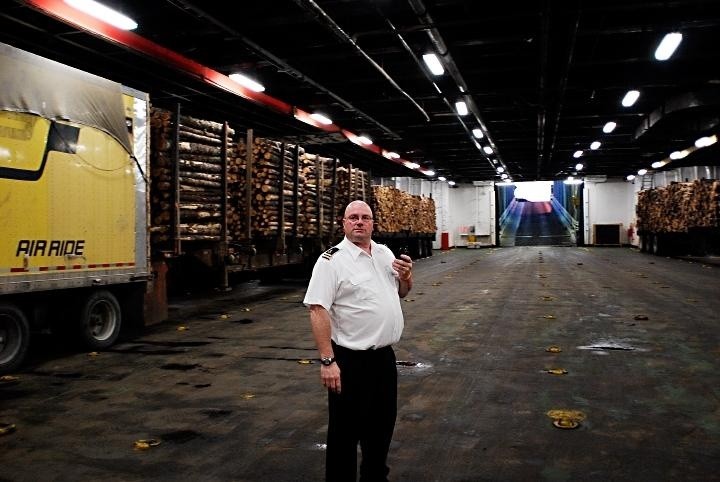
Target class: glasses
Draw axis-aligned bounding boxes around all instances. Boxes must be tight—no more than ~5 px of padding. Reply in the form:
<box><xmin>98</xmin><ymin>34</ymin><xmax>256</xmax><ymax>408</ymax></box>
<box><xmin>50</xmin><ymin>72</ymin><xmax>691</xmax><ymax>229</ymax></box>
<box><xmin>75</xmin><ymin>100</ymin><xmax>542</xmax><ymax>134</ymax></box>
<box><xmin>345</xmin><ymin>216</ymin><xmax>374</xmax><ymax>223</ymax></box>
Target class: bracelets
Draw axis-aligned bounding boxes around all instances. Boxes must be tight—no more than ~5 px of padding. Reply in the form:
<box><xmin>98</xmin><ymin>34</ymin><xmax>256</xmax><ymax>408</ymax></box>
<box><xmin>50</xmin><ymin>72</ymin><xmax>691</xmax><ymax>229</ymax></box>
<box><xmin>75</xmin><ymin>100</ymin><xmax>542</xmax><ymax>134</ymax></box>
<box><xmin>398</xmin><ymin>273</ymin><xmax>412</xmax><ymax>281</ymax></box>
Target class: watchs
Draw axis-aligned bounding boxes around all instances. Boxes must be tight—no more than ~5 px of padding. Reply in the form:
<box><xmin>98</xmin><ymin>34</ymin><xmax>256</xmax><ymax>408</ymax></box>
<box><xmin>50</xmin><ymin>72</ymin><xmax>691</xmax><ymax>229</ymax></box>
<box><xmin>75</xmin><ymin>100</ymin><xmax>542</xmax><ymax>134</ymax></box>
<box><xmin>320</xmin><ymin>356</ymin><xmax>336</xmax><ymax>366</ymax></box>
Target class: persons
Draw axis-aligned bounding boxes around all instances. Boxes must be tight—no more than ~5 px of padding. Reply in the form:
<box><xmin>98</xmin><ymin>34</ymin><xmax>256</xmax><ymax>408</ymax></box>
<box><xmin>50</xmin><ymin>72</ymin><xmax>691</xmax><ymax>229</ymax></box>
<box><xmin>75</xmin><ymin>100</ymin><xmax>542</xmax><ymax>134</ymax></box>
<box><xmin>302</xmin><ymin>200</ymin><xmax>414</xmax><ymax>482</ymax></box>
